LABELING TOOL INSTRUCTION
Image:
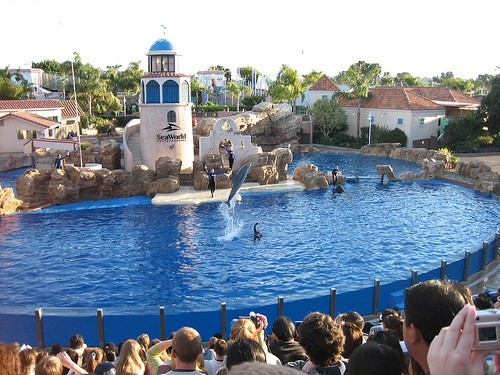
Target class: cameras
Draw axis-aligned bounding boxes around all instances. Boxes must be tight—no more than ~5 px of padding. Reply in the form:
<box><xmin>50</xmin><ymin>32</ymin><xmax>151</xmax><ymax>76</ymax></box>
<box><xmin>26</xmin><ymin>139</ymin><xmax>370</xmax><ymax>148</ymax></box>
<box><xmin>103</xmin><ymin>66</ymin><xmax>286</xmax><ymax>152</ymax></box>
<box><xmin>473</xmin><ymin>309</ymin><xmax>500</xmax><ymax>351</ymax></box>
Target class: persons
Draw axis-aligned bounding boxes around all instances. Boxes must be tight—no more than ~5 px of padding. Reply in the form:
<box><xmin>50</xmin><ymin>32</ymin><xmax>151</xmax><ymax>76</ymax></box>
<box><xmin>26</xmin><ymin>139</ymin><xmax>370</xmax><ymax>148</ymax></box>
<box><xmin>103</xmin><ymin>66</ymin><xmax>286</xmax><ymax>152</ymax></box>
<box><xmin>204</xmin><ymin>164</ymin><xmax>223</xmax><ymax>198</ymax></box>
<box><xmin>221</xmin><ymin>143</ymin><xmax>235</xmax><ymax>170</ymax></box>
<box><xmin>254</xmin><ymin>221</ymin><xmax>262</xmax><ymax>243</ymax></box>
<box><xmin>53</xmin><ymin>152</ymin><xmax>69</xmax><ymax>169</ymax></box>
<box><xmin>107</xmin><ymin>124</ymin><xmax>113</xmax><ymax>137</ymax></box>
<box><xmin>332</xmin><ymin>165</ymin><xmax>339</xmax><ymax>187</ymax></box>
<box><xmin>0</xmin><ymin>278</ymin><xmax>500</xmax><ymax>375</ymax></box>
<box><xmin>66</xmin><ymin>130</ymin><xmax>74</xmax><ymax>140</ymax></box>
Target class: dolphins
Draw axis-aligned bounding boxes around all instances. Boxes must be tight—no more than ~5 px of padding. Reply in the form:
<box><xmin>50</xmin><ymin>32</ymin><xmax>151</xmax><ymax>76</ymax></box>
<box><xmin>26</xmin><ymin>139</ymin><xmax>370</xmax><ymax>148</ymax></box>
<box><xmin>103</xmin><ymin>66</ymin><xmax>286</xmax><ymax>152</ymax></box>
<box><xmin>224</xmin><ymin>161</ymin><xmax>252</xmax><ymax>208</ymax></box>
